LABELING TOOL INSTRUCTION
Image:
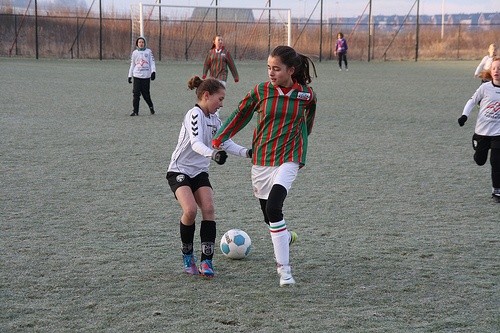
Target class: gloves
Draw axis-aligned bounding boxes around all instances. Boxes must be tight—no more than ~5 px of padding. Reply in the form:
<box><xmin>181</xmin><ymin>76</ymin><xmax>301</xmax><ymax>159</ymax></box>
<box><xmin>458</xmin><ymin>115</ymin><xmax>468</xmax><ymax>127</ymax></box>
<box><xmin>150</xmin><ymin>72</ymin><xmax>155</xmax><ymax>80</ymax></box>
<box><xmin>129</xmin><ymin>77</ymin><xmax>132</xmax><ymax>84</ymax></box>
<box><xmin>211</xmin><ymin>150</ymin><xmax>228</xmax><ymax>165</ymax></box>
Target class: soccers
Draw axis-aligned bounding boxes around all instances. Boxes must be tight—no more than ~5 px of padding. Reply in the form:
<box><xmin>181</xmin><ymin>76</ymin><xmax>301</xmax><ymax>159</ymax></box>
<box><xmin>220</xmin><ymin>228</ymin><xmax>252</xmax><ymax>259</ymax></box>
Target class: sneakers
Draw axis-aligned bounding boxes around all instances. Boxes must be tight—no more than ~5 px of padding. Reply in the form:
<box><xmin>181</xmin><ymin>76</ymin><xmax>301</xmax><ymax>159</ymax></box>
<box><xmin>182</xmin><ymin>254</ymin><xmax>198</xmax><ymax>275</ymax></box>
<box><xmin>198</xmin><ymin>258</ymin><xmax>215</xmax><ymax>276</ymax></box>
<box><xmin>290</xmin><ymin>232</ymin><xmax>297</xmax><ymax>247</ymax></box>
<box><xmin>277</xmin><ymin>265</ymin><xmax>295</xmax><ymax>287</ymax></box>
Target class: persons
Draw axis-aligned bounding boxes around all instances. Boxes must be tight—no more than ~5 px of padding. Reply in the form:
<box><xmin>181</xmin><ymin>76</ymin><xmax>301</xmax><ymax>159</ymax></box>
<box><xmin>202</xmin><ymin>34</ymin><xmax>240</xmax><ymax>118</ymax></box>
<box><xmin>210</xmin><ymin>45</ymin><xmax>318</xmax><ymax>287</ymax></box>
<box><xmin>166</xmin><ymin>75</ymin><xmax>253</xmax><ymax>276</ymax></box>
<box><xmin>458</xmin><ymin>54</ymin><xmax>500</xmax><ymax>196</ymax></box>
<box><xmin>334</xmin><ymin>32</ymin><xmax>349</xmax><ymax>71</ymax></box>
<box><xmin>473</xmin><ymin>43</ymin><xmax>498</xmax><ymax>108</ymax></box>
<box><xmin>127</xmin><ymin>36</ymin><xmax>156</xmax><ymax>116</ymax></box>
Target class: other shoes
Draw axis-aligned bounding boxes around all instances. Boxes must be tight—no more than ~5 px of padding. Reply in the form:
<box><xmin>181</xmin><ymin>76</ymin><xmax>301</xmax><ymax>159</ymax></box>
<box><xmin>150</xmin><ymin>107</ymin><xmax>154</xmax><ymax>114</ymax></box>
<box><xmin>346</xmin><ymin>69</ymin><xmax>348</xmax><ymax>71</ymax></box>
<box><xmin>339</xmin><ymin>69</ymin><xmax>342</xmax><ymax>71</ymax></box>
<box><xmin>491</xmin><ymin>192</ymin><xmax>500</xmax><ymax>203</ymax></box>
<box><xmin>130</xmin><ymin>112</ymin><xmax>138</xmax><ymax>116</ymax></box>
